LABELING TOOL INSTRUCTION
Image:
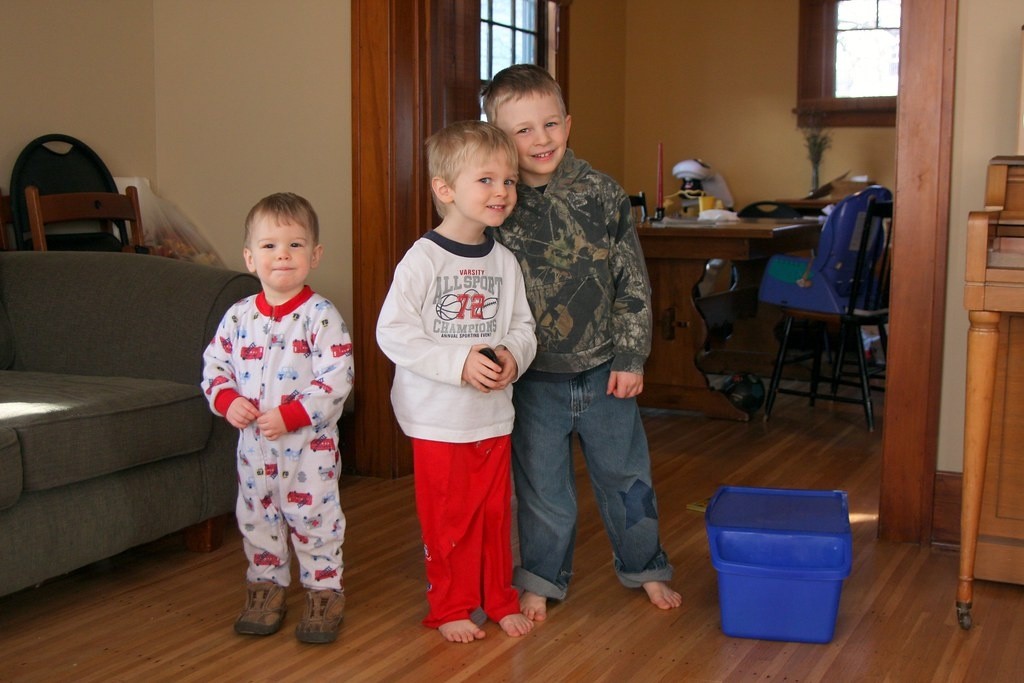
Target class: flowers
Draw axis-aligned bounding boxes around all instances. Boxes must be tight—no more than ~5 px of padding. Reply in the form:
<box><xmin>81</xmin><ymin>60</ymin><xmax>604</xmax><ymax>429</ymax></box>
<box><xmin>792</xmin><ymin>107</ymin><xmax>834</xmax><ymax>190</ymax></box>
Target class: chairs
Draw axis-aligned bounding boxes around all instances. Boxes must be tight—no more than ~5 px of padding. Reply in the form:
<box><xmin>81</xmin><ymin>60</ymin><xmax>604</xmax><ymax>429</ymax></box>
<box><xmin>629</xmin><ymin>191</ymin><xmax>647</xmax><ymax>221</ymax></box>
<box><xmin>767</xmin><ymin>196</ymin><xmax>895</xmax><ymax>433</ymax></box>
<box><xmin>693</xmin><ymin>200</ymin><xmax>818</xmax><ymax>394</ymax></box>
<box><xmin>0</xmin><ymin>134</ymin><xmax>155</xmax><ymax>255</ymax></box>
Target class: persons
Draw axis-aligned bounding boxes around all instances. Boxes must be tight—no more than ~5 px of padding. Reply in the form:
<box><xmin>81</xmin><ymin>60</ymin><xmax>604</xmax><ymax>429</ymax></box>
<box><xmin>482</xmin><ymin>65</ymin><xmax>682</xmax><ymax>623</ymax></box>
<box><xmin>376</xmin><ymin>124</ymin><xmax>539</xmax><ymax>644</ymax></box>
<box><xmin>200</xmin><ymin>193</ymin><xmax>353</xmax><ymax>644</ymax></box>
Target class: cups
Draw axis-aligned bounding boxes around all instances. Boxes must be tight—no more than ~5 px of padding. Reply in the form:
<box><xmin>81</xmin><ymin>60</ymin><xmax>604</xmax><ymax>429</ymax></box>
<box><xmin>698</xmin><ymin>196</ymin><xmax>715</xmax><ymax>213</ymax></box>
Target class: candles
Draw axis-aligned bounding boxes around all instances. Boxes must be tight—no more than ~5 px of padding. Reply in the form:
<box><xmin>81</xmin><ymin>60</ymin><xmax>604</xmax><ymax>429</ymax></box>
<box><xmin>657</xmin><ymin>141</ymin><xmax>664</xmax><ymax>208</ymax></box>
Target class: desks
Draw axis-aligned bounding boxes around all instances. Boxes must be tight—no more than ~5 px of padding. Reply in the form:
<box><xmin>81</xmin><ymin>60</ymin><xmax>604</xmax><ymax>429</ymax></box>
<box><xmin>634</xmin><ymin>219</ymin><xmax>823</xmax><ymax>423</ymax></box>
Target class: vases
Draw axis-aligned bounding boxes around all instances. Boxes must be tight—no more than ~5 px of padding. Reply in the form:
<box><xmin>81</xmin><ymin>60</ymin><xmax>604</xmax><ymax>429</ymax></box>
<box><xmin>812</xmin><ymin>164</ymin><xmax>820</xmax><ymax>189</ymax></box>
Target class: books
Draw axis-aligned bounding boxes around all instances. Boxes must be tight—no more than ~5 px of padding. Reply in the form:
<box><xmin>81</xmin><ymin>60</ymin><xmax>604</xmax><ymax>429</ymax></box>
<box><xmin>662</xmin><ymin>215</ymin><xmax>736</xmax><ymax>228</ymax></box>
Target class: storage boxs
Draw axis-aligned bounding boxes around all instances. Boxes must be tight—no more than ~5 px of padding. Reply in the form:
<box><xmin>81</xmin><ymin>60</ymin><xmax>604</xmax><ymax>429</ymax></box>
<box><xmin>830</xmin><ymin>180</ymin><xmax>876</xmax><ymax>197</ymax></box>
<box><xmin>704</xmin><ymin>486</ymin><xmax>852</xmax><ymax>643</ymax></box>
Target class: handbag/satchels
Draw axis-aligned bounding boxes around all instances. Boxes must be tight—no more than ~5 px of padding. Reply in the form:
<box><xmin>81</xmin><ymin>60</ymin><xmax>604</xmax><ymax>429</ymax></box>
<box><xmin>124</xmin><ymin>176</ymin><xmax>228</xmax><ymax>269</ymax></box>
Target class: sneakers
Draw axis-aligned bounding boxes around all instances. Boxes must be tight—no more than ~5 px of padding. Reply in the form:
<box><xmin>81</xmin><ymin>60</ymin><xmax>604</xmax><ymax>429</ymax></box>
<box><xmin>235</xmin><ymin>582</ymin><xmax>287</xmax><ymax>635</ymax></box>
<box><xmin>295</xmin><ymin>589</ymin><xmax>345</xmax><ymax>643</ymax></box>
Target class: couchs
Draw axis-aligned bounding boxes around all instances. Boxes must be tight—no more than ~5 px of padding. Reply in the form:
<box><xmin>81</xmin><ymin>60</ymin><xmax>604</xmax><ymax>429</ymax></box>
<box><xmin>0</xmin><ymin>251</ymin><xmax>261</xmax><ymax>601</ymax></box>
